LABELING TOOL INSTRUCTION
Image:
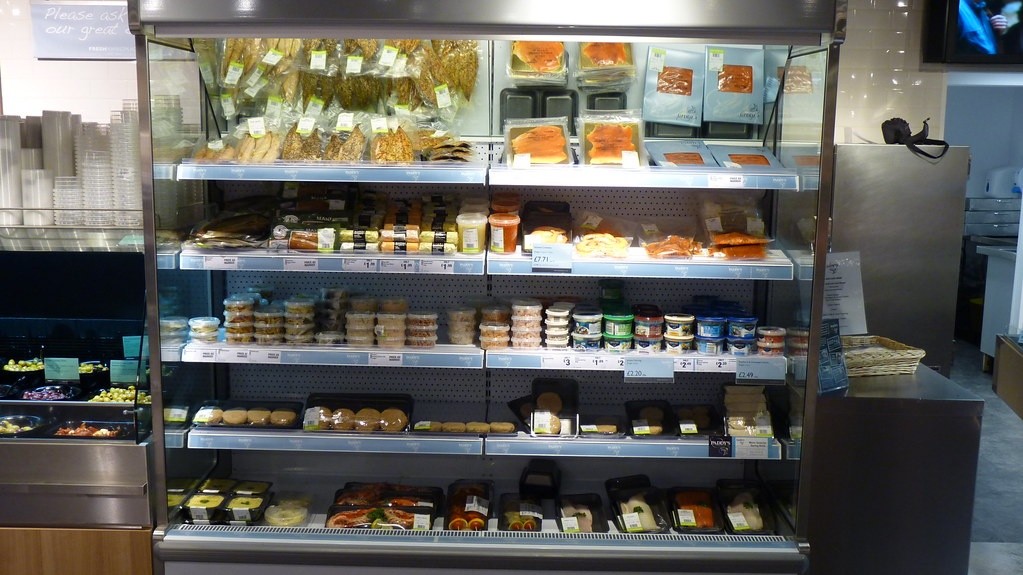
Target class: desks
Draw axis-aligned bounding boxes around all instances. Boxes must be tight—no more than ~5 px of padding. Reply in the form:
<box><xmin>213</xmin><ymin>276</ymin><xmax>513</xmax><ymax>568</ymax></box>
<box><xmin>809</xmin><ymin>339</ymin><xmax>988</xmax><ymax>575</ymax></box>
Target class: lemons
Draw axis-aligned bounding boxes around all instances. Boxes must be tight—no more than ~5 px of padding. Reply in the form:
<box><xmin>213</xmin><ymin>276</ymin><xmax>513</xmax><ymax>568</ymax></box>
<box><xmin>372</xmin><ymin>519</ymin><xmax>382</xmax><ymax>529</ymax></box>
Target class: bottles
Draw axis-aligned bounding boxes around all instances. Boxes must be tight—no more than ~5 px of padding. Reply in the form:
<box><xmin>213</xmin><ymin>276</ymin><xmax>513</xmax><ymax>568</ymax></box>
<box><xmin>161</xmin><ymin>295</ymin><xmax>810</xmax><ymax>357</ymax></box>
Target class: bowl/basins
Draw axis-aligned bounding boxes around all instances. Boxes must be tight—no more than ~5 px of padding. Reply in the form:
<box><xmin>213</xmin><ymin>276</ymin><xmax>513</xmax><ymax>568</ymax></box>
<box><xmin>0</xmin><ymin>357</ymin><xmax>110</xmax><ymax>436</ymax></box>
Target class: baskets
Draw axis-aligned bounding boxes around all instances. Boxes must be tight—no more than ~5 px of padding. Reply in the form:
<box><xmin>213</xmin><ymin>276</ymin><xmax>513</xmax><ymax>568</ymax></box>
<box><xmin>838</xmin><ymin>336</ymin><xmax>926</xmax><ymax>376</ymax></box>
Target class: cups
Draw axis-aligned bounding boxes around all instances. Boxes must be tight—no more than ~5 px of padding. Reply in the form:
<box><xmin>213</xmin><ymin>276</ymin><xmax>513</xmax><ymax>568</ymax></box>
<box><xmin>456</xmin><ymin>192</ymin><xmax>520</xmax><ymax>255</ymax></box>
<box><xmin>0</xmin><ymin>95</ymin><xmax>202</xmax><ymax>226</ymax></box>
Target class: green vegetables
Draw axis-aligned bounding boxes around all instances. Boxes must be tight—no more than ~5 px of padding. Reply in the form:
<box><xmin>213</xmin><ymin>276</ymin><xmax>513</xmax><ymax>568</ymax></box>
<box><xmin>367</xmin><ymin>507</ymin><xmax>388</xmax><ymax>522</ymax></box>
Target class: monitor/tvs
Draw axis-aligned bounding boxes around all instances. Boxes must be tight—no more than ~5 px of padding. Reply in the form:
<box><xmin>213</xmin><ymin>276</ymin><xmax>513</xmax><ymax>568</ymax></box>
<box><xmin>918</xmin><ymin>0</ymin><xmax>1023</xmax><ymax>74</ymax></box>
